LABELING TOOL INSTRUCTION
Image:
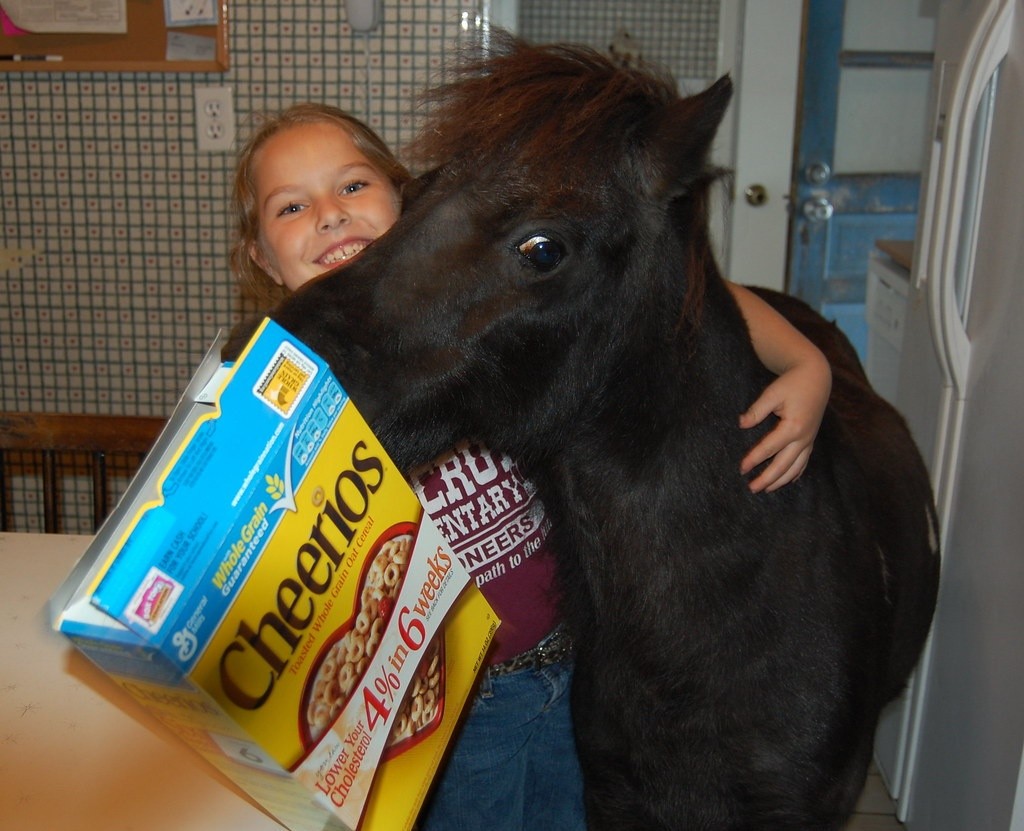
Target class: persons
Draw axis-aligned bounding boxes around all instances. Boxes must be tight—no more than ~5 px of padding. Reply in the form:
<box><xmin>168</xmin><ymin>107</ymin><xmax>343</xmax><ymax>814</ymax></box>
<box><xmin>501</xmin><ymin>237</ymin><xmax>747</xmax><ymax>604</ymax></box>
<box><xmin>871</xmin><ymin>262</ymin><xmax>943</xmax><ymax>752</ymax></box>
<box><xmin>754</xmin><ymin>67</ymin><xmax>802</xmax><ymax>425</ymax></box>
<box><xmin>228</xmin><ymin>103</ymin><xmax>833</xmax><ymax>831</ymax></box>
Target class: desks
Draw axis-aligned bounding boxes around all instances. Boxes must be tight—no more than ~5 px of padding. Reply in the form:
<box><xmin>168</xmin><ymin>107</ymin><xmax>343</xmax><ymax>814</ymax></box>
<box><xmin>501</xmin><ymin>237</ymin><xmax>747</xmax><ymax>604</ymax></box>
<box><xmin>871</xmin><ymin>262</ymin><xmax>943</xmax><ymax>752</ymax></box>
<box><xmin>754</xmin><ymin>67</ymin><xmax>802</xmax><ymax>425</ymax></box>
<box><xmin>0</xmin><ymin>532</ymin><xmax>291</xmax><ymax>831</ymax></box>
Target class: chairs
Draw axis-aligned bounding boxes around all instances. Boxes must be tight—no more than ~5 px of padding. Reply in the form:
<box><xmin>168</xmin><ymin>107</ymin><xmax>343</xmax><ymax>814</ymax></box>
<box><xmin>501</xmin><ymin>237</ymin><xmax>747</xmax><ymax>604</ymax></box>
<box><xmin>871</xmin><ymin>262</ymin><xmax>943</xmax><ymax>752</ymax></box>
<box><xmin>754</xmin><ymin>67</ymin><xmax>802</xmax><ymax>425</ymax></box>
<box><xmin>0</xmin><ymin>411</ymin><xmax>171</xmax><ymax>535</ymax></box>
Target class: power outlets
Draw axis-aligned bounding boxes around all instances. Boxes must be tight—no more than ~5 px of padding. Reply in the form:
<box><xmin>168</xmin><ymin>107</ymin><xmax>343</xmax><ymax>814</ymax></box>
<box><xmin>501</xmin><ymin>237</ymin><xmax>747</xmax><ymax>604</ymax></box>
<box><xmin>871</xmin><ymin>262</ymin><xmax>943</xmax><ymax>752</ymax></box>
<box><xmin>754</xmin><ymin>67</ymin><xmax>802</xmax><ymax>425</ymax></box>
<box><xmin>194</xmin><ymin>85</ymin><xmax>236</xmax><ymax>152</ymax></box>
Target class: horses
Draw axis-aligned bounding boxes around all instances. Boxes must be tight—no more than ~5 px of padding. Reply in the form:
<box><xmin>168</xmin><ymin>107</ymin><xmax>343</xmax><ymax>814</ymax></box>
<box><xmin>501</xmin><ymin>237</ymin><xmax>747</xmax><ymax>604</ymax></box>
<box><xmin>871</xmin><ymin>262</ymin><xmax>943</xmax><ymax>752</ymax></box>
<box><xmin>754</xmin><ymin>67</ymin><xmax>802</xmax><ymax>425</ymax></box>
<box><xmin>219</xmin><ymin>22</ymin><xmax>944</xmax><ymax>831</ymax></box>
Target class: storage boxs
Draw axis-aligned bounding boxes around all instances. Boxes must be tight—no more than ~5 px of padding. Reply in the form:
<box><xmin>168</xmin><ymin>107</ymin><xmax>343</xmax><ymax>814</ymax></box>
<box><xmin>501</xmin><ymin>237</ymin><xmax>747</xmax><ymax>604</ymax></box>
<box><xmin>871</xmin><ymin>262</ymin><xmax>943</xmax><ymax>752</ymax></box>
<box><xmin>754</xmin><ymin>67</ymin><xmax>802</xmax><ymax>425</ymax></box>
<box><xmin>41</xmin><ymin>318</ymin><xmax>502</xmax><ymax>831</ymax></box>
